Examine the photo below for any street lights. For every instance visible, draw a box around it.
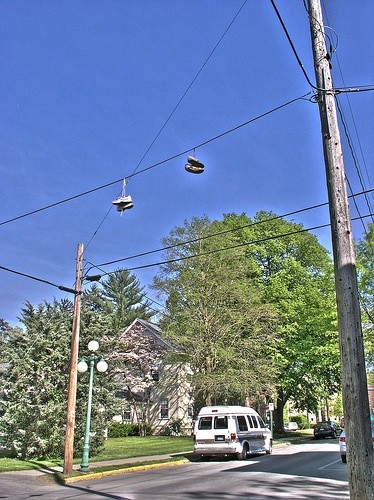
[77,339,108,472]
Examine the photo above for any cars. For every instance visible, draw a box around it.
[313,415,345,439]
[265,420,275,429]
[283,421,299,431]
[339,416,374,463]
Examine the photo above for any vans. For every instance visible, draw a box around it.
[193,405,274,462]
